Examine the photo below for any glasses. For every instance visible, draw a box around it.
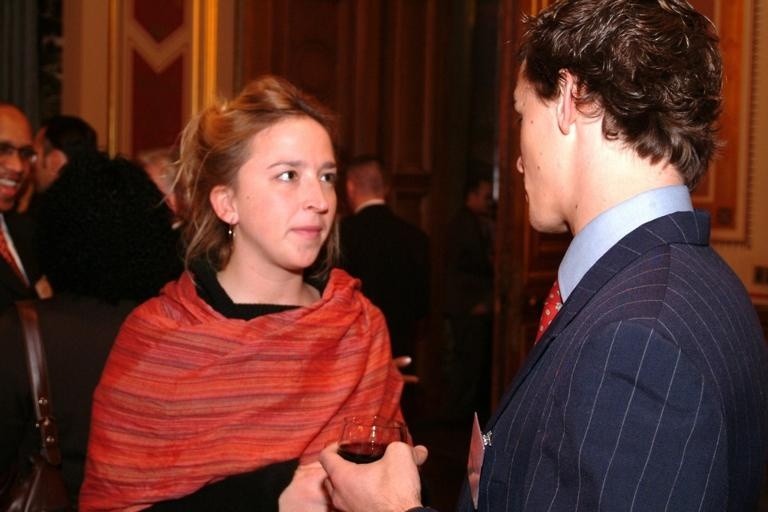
[0,140,41,166]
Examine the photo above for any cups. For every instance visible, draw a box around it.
[337,414,408,466]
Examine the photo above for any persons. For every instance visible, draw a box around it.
[307,154,434,403]
[0,100,205,512]
[316,0,765,512]
[77,76,415,512]
[441,182,495,431]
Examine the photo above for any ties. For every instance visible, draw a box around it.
[0,220,26,288]
[533,268,564,350]
